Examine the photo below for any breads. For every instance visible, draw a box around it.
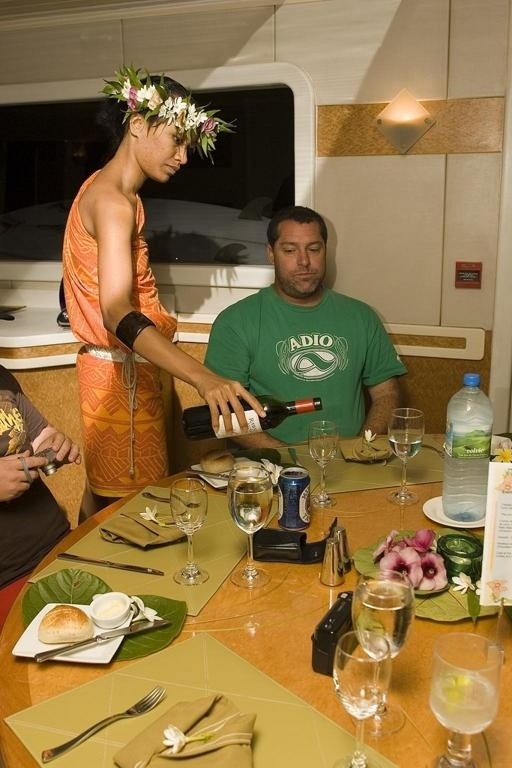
[38,604,93,643]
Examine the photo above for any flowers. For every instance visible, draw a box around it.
[99,62,240,166]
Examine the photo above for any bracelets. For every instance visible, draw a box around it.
[116,310,156,350]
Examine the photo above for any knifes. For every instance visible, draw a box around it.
[183,467,268,483]
[57,549,164,580]
[32,615,172,663]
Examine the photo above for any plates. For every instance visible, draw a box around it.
[422,493,484,530]
[10,601,135,665]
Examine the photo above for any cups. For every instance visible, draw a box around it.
[388,409,425,507]
[168,477,210,588]
[350,567,416,738]
[307,419,339,510]
[425,631,504,768]
[226,460,276,592]
[330,629,394,768]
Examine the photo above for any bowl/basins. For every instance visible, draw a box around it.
[86,591,133,629]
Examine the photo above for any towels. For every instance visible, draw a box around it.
[338,438,392,464]
[100,512,191,552]
[115,697,256,768]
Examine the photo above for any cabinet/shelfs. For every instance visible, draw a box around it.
[0,304,179,530]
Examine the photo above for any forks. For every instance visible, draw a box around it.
[40,684,170,764]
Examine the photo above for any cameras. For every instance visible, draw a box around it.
[312,590,354,676]
[34,448,71,476]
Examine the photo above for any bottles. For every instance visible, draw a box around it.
[182,395,322,440]
[320,538,344,587]
[442,374,493,521]
[334,527,351,573]
[278,467,312,531]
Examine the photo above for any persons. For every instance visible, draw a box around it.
[63,75,266,527]
[204,207,409,451]
[0,363,82,632]
